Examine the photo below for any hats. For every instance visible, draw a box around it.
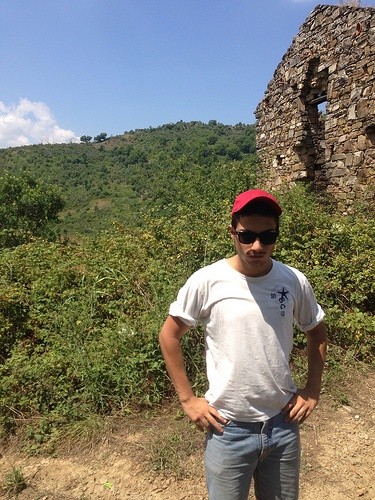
[230,190,282,216]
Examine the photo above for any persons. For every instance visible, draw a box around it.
[159,190,328,500]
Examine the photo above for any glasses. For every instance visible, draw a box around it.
[235,230,278,245]
